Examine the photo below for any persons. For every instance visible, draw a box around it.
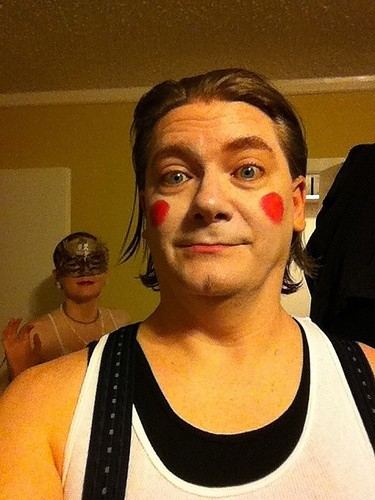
[0,232,132,385]
[0,69,375,500]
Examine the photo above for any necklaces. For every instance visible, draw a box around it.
[60,298,105,345]
